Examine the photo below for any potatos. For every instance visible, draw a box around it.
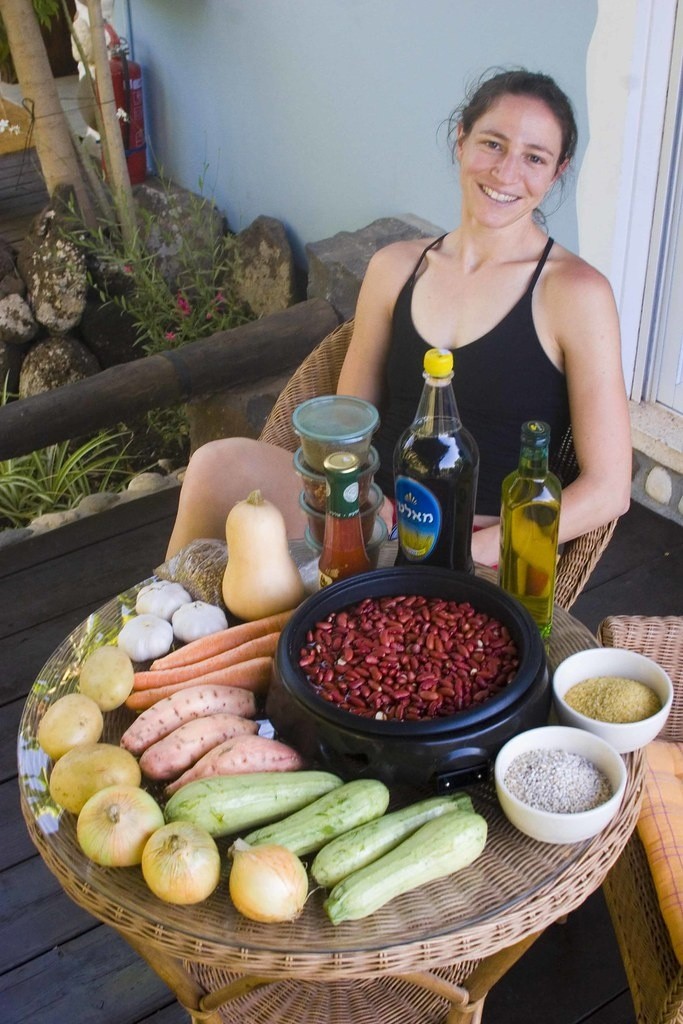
[122,684,300,794]
[39,647,141,813]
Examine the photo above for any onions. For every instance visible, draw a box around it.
[77,785,309,923]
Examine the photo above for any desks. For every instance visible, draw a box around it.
[12,530,644,1024]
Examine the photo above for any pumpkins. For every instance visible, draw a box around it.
[222,489,303,622]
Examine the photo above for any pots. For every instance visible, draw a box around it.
[264,566,551,812]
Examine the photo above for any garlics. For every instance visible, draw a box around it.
[117,580,227,661]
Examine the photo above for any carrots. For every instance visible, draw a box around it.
[126,609,295,710]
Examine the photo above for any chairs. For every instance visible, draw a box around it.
[593,614,683,1024]
[249,311,618,613]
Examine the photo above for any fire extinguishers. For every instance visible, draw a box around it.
[100,17,147,188]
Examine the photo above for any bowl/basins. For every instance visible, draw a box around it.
[298,483,383,546]
[291,395,379,472]
[552,647,674,753]
[293,445,378,512]
[494,726,628,844]
[304,514,388,571]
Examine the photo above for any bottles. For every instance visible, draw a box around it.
[394,349,479,571]
[314,452,372,591]
[498,421,563,636]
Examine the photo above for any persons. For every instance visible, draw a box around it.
[163,65,635,576]
